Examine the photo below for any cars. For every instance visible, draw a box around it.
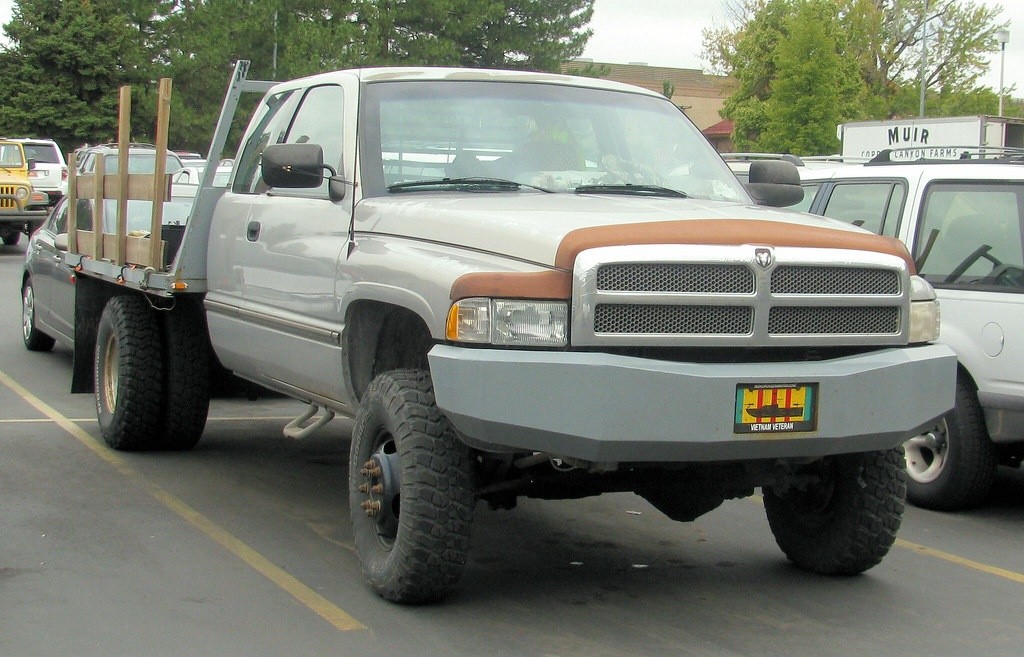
[0,133,236,247]
[18,183,202,354]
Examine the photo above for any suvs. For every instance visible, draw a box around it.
[712,149,1023,514]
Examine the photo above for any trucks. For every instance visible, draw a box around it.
[835,113,1024,162]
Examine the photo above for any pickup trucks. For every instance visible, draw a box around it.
[59,57,961,608]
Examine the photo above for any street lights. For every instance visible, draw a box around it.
[996,28,1010,117]
